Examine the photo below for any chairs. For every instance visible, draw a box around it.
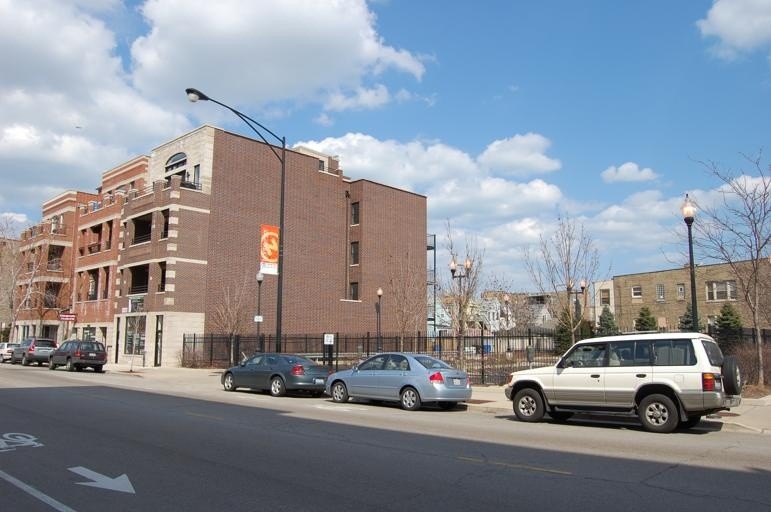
[373,356,410,370]
[588,345,650,366]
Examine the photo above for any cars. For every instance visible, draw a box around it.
[0,337,107,373]
[221,352,472,411]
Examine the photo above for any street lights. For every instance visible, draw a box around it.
[377,286,383,352]
[504,293,512,352]
[256,272,264,354]
[185,88,286,352]
[451,258,471,334]
[567,280,585,341]
[681,194,699,332]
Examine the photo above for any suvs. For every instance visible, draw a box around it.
[505,332,742,433]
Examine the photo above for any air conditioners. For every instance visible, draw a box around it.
[676,295,684,301]
